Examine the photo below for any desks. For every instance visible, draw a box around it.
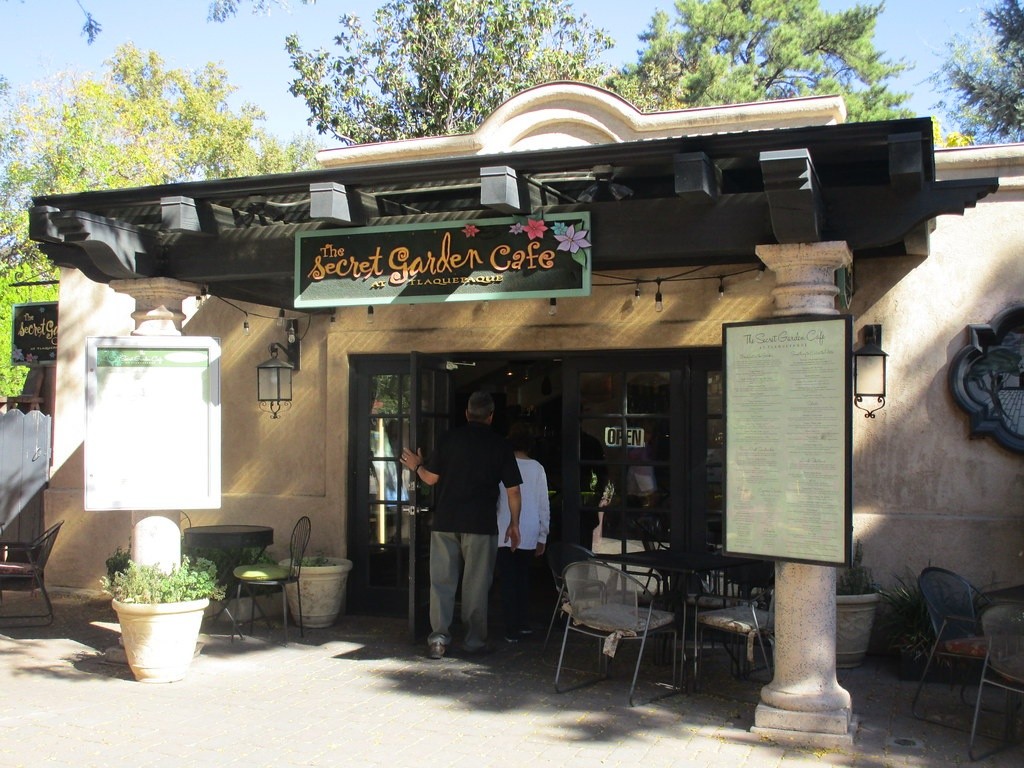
[587,548,762,697]
[185,523,274,639]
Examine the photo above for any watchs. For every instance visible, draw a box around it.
[415,464,422,471]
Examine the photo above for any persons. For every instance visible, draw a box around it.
[546,396,607,553]
[497,423,550,640]
[630,401,670,506]
[400,392,523,659]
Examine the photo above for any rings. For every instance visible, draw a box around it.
[405,458,407,460]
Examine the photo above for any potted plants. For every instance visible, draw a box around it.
[100,548,228,682]
[881,558,943,682]
[279,556,352,628]
[835,542,880,670]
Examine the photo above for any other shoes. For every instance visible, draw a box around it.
[502,629,519,643]
[472,639,496,659]
[518,624,534,635]
[429,641,449,658]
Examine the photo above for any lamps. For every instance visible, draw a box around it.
[256,338,300,419]
[852,324,888,417]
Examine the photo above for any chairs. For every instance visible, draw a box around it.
[217,515,312,647]
[0,518,66,629]
[544,540,775,708]
[911,567,1024,768]
